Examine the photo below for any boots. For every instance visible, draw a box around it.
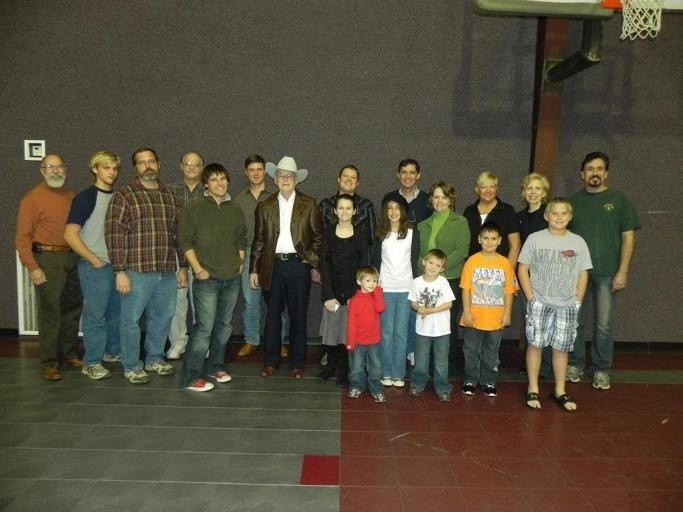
[318,343,348,388]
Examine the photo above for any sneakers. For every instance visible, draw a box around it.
[462,383,477,396]
[479,382,497,397]
[592,370,611,390]
[166,347,186,359]
[565,364,585,383]
[185,379,215,393]
[43,353,176,384]
[345,375,451,403]
[209,370,232,383]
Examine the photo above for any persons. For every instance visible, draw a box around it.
[105,148,175,384]
[464,171,523,369]
[517,196,593,411]
[567,152,642,389]
[65,151,124,380]
[407,249,456,403]
[415,181,471,381]
[236,154,435,404]
[166,151,211,360]
[176,164,247,391]
[13,153,83,381]
[458,222,520,397]
[515,172,552,379]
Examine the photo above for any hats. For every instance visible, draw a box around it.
[265,155,309,184]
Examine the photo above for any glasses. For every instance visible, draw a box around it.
[43,164,67,171]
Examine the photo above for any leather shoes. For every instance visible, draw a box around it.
[291,367,304,378]
[262,366,275,377]
[280,345,288,358]
[237,343,257,357]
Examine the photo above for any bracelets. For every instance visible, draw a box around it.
[195,270,205,275]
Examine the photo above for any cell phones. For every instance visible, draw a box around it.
[324,301,340,313]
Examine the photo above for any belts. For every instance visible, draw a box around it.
[31,242,70,253]
[273,252,301,261]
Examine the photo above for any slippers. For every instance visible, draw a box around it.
[525,390,543,410]
[553,391,576,413]
